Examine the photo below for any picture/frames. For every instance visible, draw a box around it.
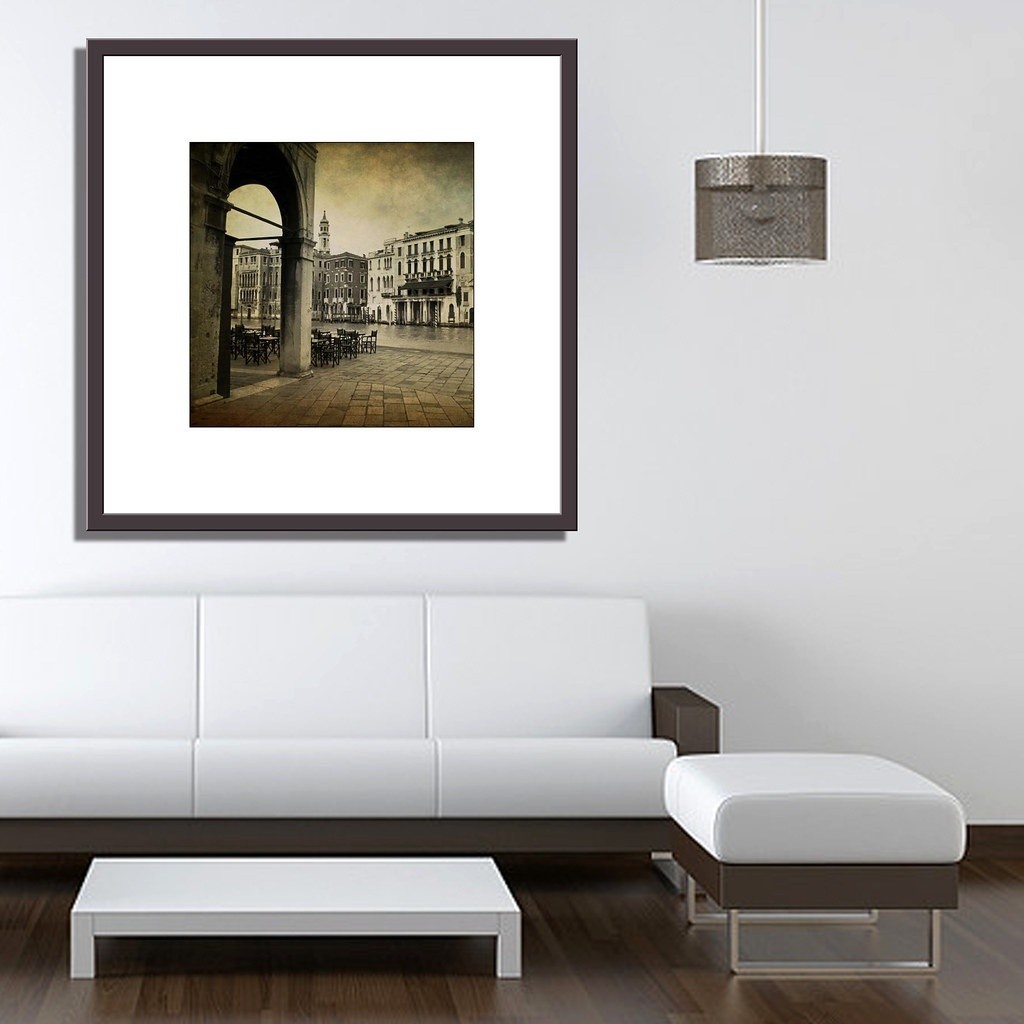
[85,38,583,533]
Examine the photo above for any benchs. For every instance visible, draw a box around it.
[0,592,722,898]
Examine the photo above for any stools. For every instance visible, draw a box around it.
[664,751,968,976]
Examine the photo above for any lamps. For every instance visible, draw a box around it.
[696,0,830,265]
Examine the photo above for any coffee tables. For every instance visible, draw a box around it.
[67,856,523,980]
